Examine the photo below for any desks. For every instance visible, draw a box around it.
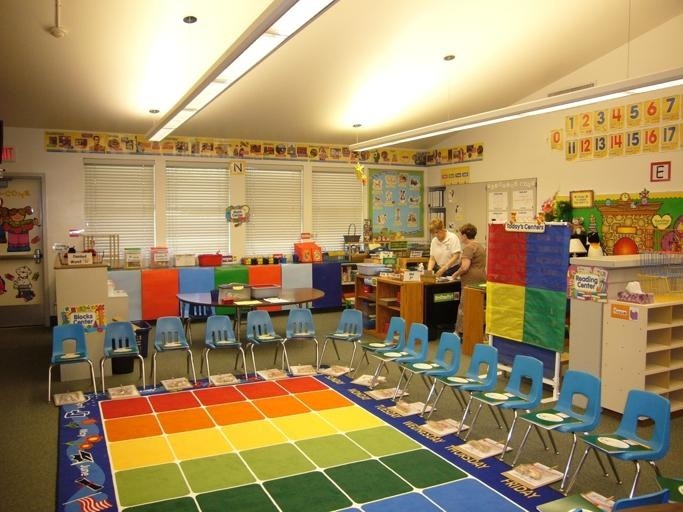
[176,287,325,346]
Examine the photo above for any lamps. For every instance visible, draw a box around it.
[349,0,683,152]
[146,0,338,141]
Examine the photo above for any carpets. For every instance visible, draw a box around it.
[55,366,611,512]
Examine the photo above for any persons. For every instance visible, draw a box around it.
[453,224,486,337]
[427,216,461,277]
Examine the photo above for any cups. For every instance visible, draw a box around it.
[210,290,219,302]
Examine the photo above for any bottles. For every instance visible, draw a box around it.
[418,262,424,273]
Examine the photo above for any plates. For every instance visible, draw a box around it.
[437,276,460,281]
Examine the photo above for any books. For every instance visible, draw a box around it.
[419,418,513,461]
[55,351,87,363]
[500,462,564,490]
[361,340,414,363]
[350,374,387,388]
[158,342,188,352]
[256,334,284,343]
[327,333,355,340]
[470,391,583,430]
[160,376,194,392]
[109,346,140,356]
[320,364,355,377]
[108,384,141,399]
[363,387,409,401]
[256,368,289,382]
[289,364,317,376]
[377,401,438,418]
[53,391,86,408]
[215,340,242,347]
[291,332,313,337]
[208,373,241,386]
[579,433,655,454]
[536,492,614,512]
[398,359,484,387]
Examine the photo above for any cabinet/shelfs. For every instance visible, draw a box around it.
[604,301,683,421]
[0,171,51,328]
[354,276,424,341]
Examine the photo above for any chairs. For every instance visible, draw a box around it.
[320,309,369,372]
[244,309,292,379]
[564,389,673,499]
[48,316,196,405]
[283,307,320,370]
[399,324,605,512]
[612,488,669,512]
[199,315,248,384]
[353,315,409,391]
